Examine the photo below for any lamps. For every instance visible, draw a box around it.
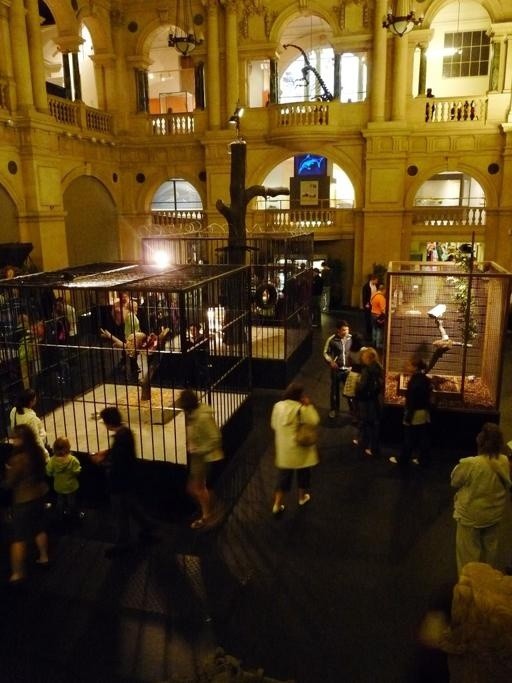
[382,0,424,37]
[168,0,205,56]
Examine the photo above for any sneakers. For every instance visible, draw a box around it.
[2,509,162,588]
[328,407,426,468]
[187,491,310,536]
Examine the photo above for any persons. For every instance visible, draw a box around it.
[323,322,450,467]
[271,385,320,513]
[13,298,247,405]
[93,408,156,559]
[425,87,436,122]
[249,260,330,328]
[362,275,387,349]
[2,388,82,585]
[419,422,511,681]
[176,390,223,532]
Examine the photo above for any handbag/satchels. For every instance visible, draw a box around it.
[292,405,318,447]
